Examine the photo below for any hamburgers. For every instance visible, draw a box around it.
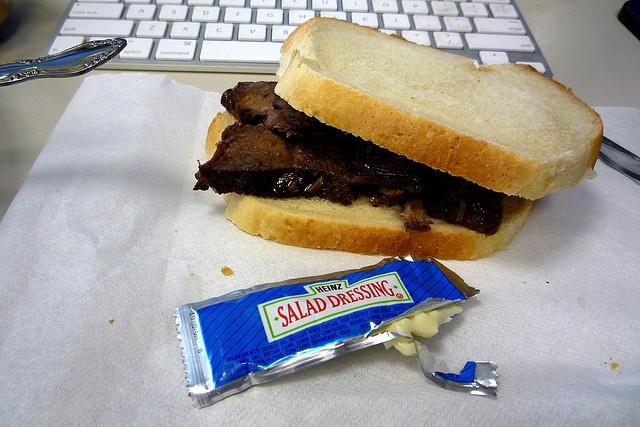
[195,16,602,258]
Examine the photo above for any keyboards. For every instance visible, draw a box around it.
[42,0,553,80]
